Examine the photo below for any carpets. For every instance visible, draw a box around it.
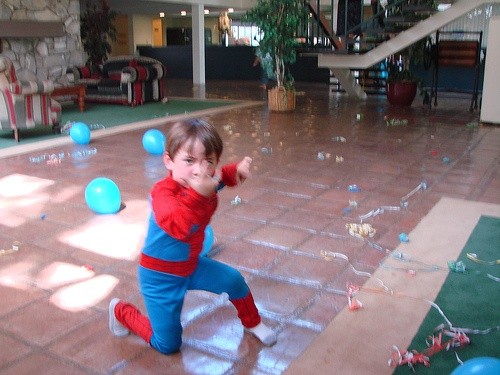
[0,96,267,158]
[281,195,500,375]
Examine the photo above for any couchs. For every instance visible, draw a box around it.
[72,56,167,107]
[0,54,62,143]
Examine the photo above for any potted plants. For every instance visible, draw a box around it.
[372,0,437,108]
[241,0,311,112]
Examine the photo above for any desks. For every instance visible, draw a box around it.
[50,85,87,112]
